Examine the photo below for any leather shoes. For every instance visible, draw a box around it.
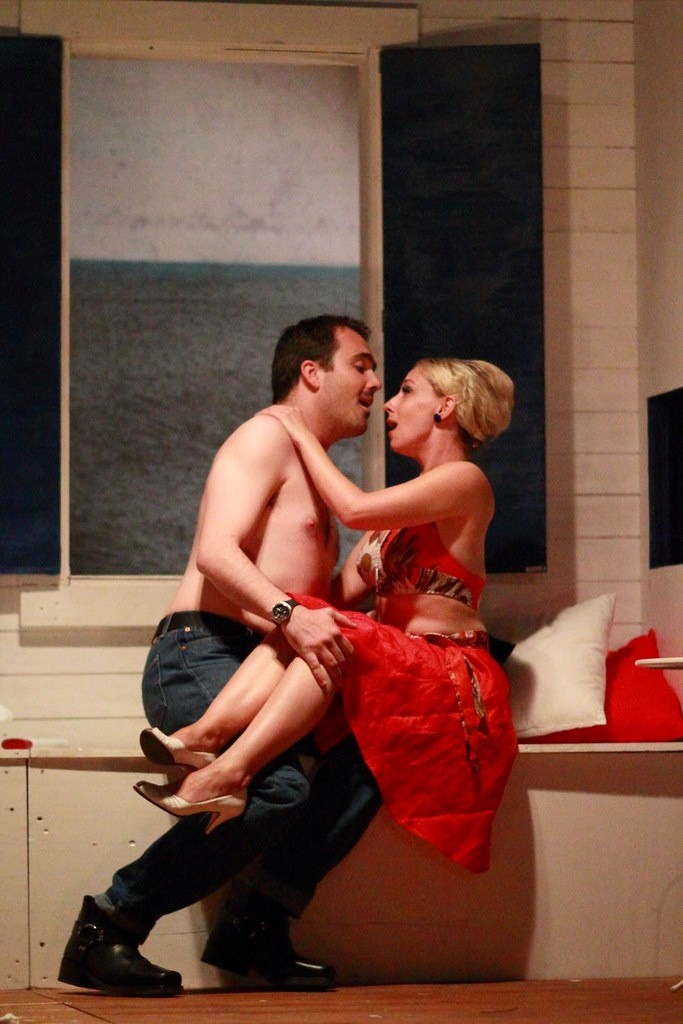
[200,880,337,988]
[57,895,185,995]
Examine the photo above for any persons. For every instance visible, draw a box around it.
[132,353,514,869]
[57,315,383,997]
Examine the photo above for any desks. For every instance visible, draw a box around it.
[0,744,683,991]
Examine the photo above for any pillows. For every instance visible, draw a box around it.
[521,628,683,744]
[503,592,615,739]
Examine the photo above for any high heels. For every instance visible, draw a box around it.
[133,780,247,834]
[140,726,219,771]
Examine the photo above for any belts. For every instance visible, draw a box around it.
[155,611,252,647]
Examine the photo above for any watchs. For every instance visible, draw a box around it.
[272,598,298,627]
